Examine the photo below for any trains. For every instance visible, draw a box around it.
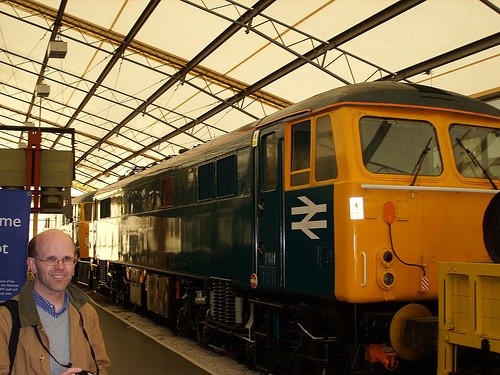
[41,81,499,375]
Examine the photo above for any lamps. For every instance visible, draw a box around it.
[18,135,27,149]
[46,23,67,59]
[35,70,50,97]
[25,112,35,133]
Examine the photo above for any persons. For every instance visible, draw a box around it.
[0,228,111,375]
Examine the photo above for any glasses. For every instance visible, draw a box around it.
[34,257,78,266]
